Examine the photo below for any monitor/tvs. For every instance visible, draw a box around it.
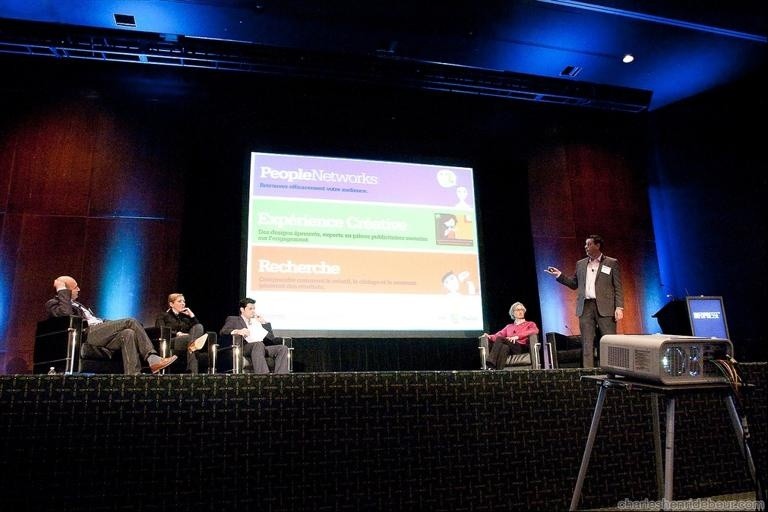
[653,295,732,342]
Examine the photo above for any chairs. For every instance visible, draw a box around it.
[34,319,293,374]
[477,333,556,370]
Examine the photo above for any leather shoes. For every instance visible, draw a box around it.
[150,355,177,374]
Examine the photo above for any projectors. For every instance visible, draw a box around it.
[599,331,737,386]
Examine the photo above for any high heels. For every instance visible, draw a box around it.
[188,333,208,355]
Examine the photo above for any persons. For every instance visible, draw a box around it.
[477,301,539,370]
[542,234,625,368]
[219,297,289,375]
[46,275,178,375]
[156,292,210,375]
